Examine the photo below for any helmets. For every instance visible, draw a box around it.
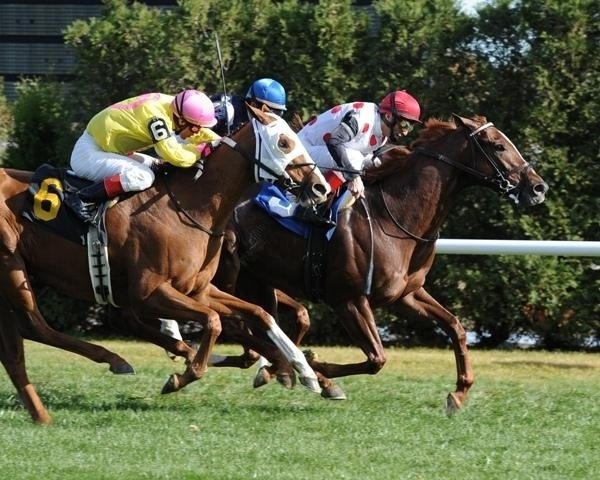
[379,90,422,123]
[246,78,287,111]
[172,90,218,128]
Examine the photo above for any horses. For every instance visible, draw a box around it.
[0,105,553,423]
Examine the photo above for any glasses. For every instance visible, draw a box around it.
[395,117,414,133]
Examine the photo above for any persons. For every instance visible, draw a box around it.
[62,89,219,220]
[296,91,422,218]
[163,76,287,181]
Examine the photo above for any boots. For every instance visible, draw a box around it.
[64,178,109,228]
[292,188,335,225]
